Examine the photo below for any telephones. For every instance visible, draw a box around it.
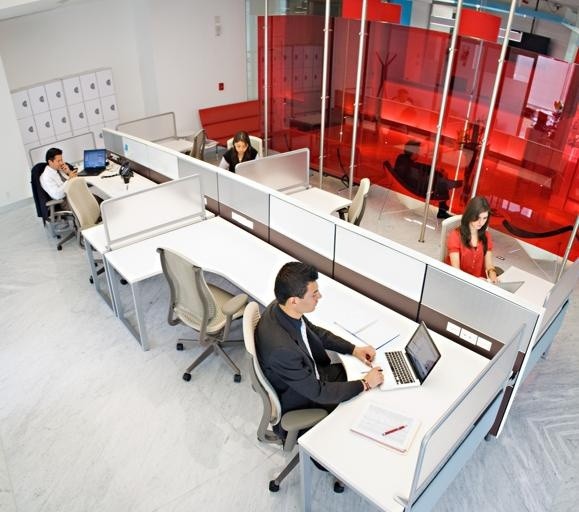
[119,160,134,177]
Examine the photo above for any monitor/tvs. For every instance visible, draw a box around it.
[505,29,550,69]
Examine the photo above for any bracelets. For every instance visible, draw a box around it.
[362,378,371,391]
[487,268,497,276]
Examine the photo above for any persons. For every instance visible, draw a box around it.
[446,197,501,284]
[254,262,384,472]
[393,141,463,218]
[391,89,414,105]
[218,131,261,173]
[39,148,79,221]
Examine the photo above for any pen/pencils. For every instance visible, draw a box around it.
[362,369,383,374]
[382,425,408,436]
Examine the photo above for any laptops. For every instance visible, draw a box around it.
[371,321,441,391]
[77,149,106,176]
[490,280,524,293]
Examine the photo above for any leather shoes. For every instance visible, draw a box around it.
[449,180,462,188]
[437,210,452,219]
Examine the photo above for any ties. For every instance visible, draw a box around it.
[301,320,320,380]
[57,170,67,182]
